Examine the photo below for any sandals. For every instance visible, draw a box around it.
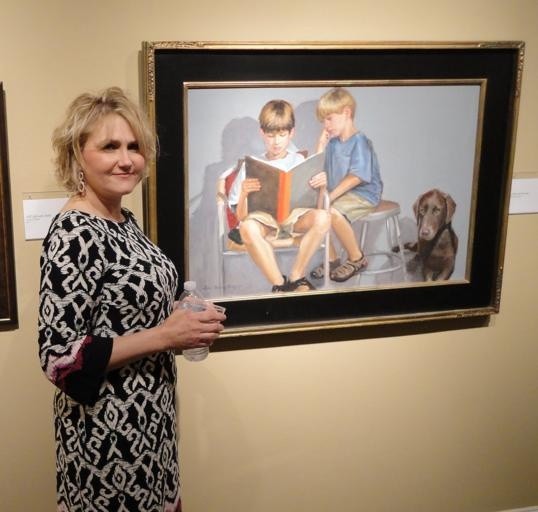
[330,253,368,282]
[272,273,315,293]
[311,259,340,279]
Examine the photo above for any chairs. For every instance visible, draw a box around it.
[214,160,331,297]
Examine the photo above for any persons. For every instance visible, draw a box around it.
[36,84,226,511]
[309,87,384,283]
[227,98,332,294]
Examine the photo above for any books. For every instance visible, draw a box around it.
[242,150,327,226]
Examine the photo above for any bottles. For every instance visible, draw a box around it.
[179,282,212,360]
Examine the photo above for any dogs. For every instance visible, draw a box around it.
[391,188,459,282]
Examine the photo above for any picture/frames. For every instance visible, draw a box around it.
[142,40,525,355]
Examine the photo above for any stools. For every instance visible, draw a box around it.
[339,201,408,286]
